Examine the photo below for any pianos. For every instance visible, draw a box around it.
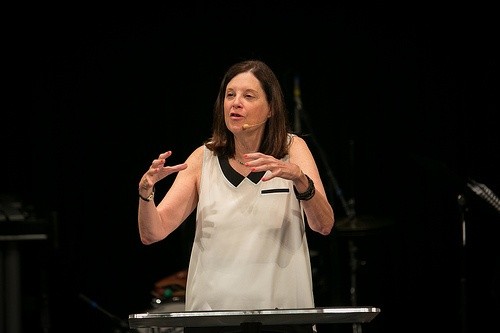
[128,306,381,328]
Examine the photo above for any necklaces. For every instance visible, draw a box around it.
[227,152,244,165]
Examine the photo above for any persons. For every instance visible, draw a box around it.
[137,58,335,333]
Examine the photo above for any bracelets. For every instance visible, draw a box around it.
[293,174,316,201]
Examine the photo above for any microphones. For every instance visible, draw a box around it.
[243,118,267,128]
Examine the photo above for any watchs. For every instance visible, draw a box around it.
[139,187,156,202]
